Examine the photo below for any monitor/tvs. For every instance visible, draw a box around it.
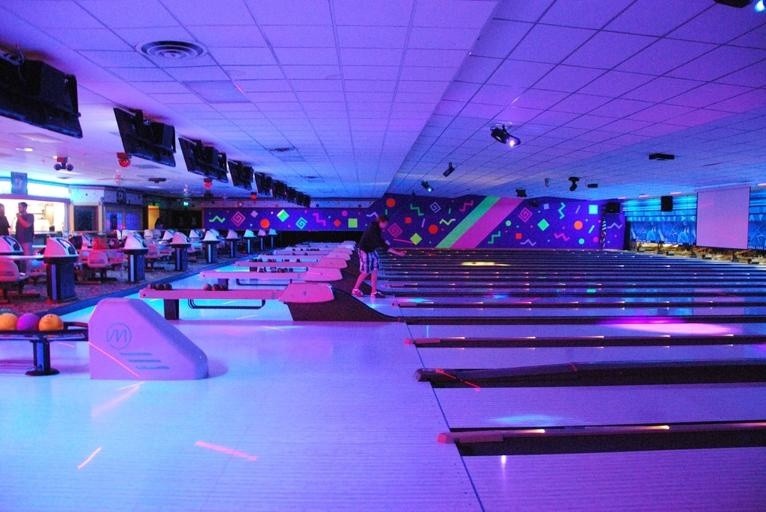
[177,135,228,184]
[112,105,177,170]
[227,157,312,210]
[0,51,84,140]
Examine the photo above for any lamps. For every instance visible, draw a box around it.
[568,176,579,191]
[420,180,434,193]
[491,124,521,147]
[443,162,455,177]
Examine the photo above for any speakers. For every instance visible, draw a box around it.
[661,196,673,212]
[606,202,621,214]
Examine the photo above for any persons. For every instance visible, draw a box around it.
[0,203,11,235]
[351,214,407,299]
[16,201,35,273]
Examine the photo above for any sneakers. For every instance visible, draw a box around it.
[351,288,364,296]
[370,290,386,298]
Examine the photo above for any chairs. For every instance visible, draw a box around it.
[0,229,246,299]
